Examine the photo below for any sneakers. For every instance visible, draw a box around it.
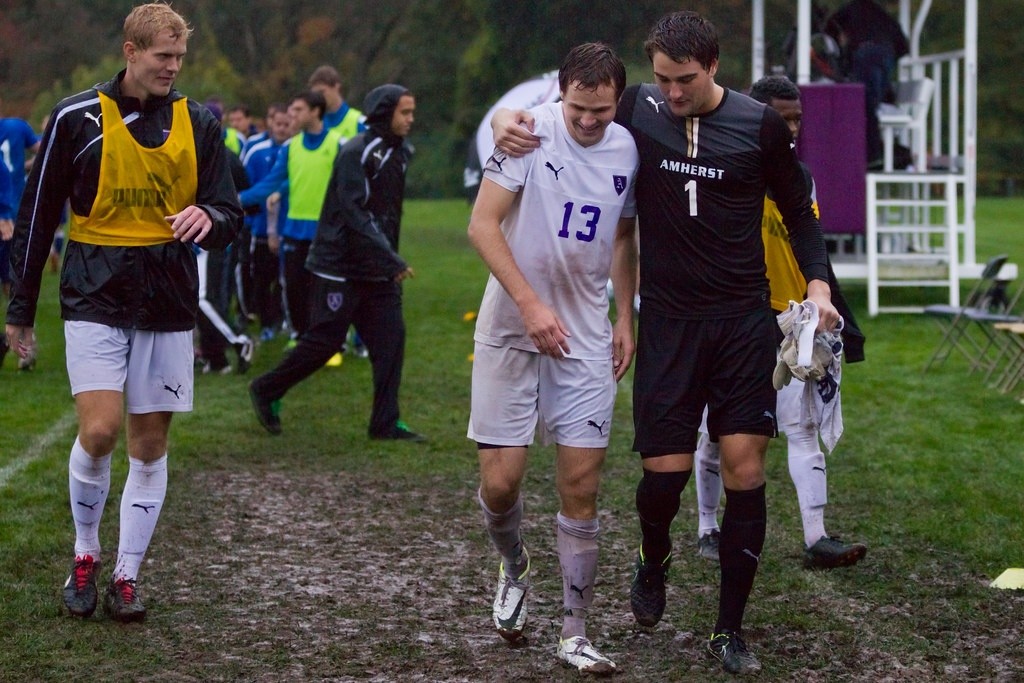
[493,548,531,640]
[803,535,865,570]
[697,528,722,563]
[249,377,283,434]
[103,575,146,621]
[556,635,618,676]
[631,545,672,628]
[63,559,98,616]
[706,629,763,677]
[371,420,429,443]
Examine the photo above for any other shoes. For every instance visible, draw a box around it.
[188,321,370,377]
[18,334,38,374]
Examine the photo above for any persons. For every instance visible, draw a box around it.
[0,115,67,373]
[249,85,422,438]
[489,12,842,679]
[467,43,642,675]
[691,73,870,569]
[194,67,372,379]
[5,0,246,619]
[824,0,908,169]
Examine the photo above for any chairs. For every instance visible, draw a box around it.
[873,78,934,170]
[916,252,1009,376]
[990,319,1024,396]
[964,274,1024,383]
